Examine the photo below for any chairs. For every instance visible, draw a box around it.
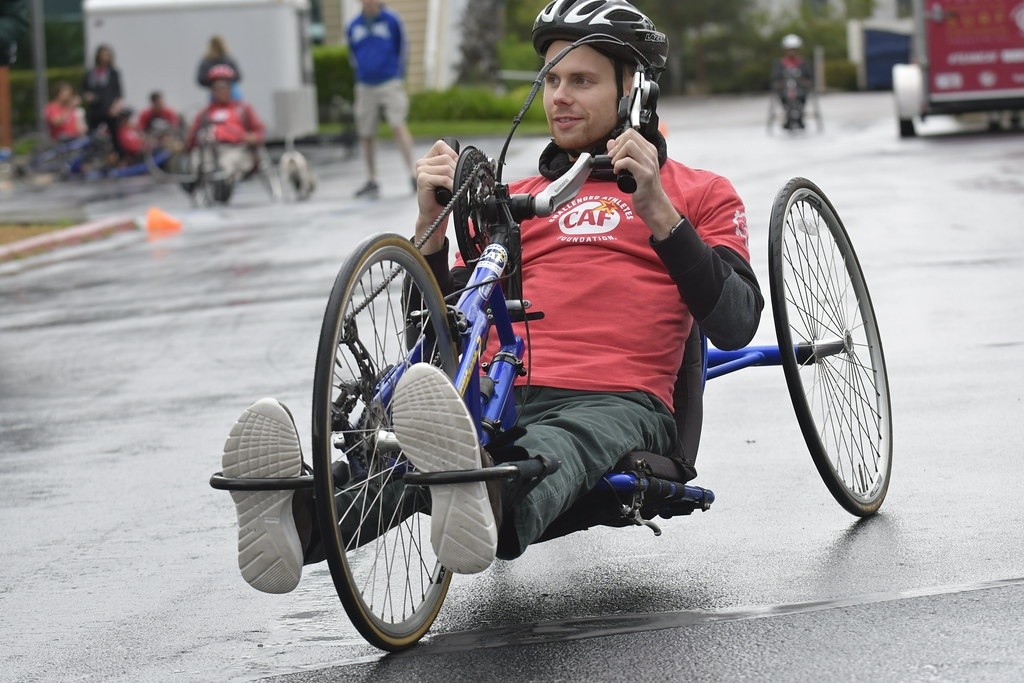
[539,315,712,543]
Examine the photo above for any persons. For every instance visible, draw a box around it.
[117,111,157,155]
[776,35,813,129]
[82,45,127,165]
[198,37,242,102]
[45,82,88,144]
[347,0,420,196]
[222,0,765,596]
[180,63,266,202]
[136,94,181,134]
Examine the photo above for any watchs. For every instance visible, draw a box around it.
[672,219,684,235]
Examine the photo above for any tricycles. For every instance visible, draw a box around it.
[761,68,826,135]
[147,111,316,208]
[204,33,890,655]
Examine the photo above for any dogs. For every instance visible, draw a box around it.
[276,132,316,204]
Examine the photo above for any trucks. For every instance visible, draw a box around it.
[893,0,1024,136]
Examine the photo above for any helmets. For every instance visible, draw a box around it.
[533,0,670,84]
[208,65,236,80]
[780,35,804,49]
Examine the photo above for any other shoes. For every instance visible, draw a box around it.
[392,363,507,575]
[222,399,317,594]
[354,181,380,196]
[411,177,417,192]
